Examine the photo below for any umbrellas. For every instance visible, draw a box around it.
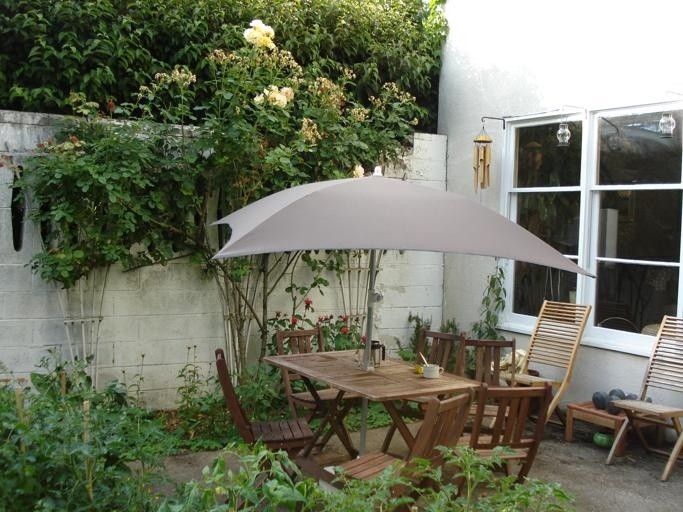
[208,166,599,367]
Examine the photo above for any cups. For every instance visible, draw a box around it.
[423,364,445,378]
[371,340,385,368]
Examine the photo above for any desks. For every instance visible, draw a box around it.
[565,403,666,456]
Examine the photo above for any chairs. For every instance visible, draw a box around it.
[215,348,314,512]
[275,323,359,458]
[336,386,473,497]
[455,380,551,498]
[464,337,516,418]
[380,330,466,454]
[607,315,683,481]
[492,299,592,424]
